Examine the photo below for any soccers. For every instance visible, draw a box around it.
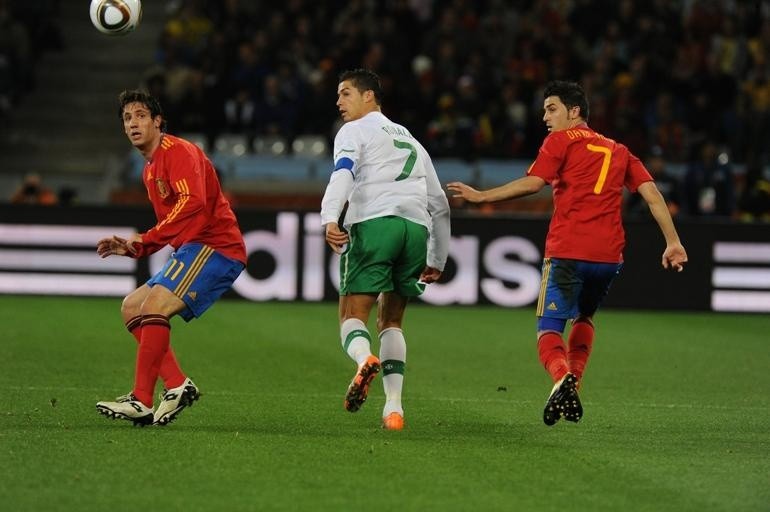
[90,0,142,36]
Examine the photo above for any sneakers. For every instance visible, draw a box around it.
[152,378,199,427]
[345,355,381,413]
[96,391,154,427]
[563,387,583,424]
[382,412,403,429]
[543,372,579,426]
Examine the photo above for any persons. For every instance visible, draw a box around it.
[318,69,450,429]
[95,91,249,426]
[1,0,770,226]
[445,80,688,426]
